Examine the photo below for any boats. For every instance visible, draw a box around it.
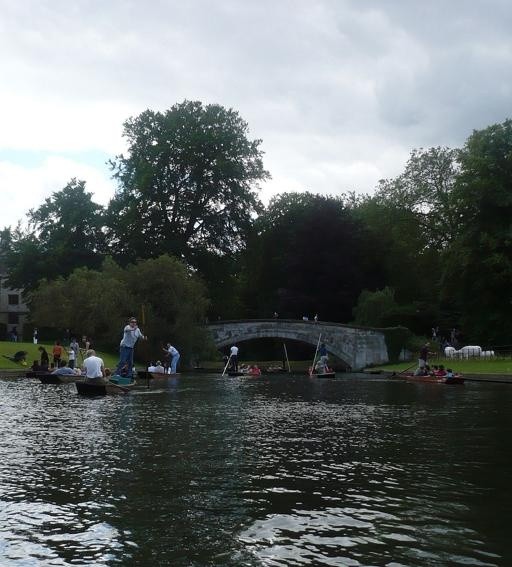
[405,374,464,384]
[26,370,182,396]
[226,365,335,378]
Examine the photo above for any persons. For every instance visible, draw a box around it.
[115,317,148,378]
[53,342,61,367]
[312,343,333,374]
[148,358,172,373]
[83,337,112,384]
[223,353,261,375]
[314,314,318,321]
[117,362,136,376]
[10,327,19,342]
[416,364,457,377]
[162,342,181,374]
[65,338,79,368]
[229,342,239,374]
[431,320,465,352]
[38,347,50,372]
[273,311,279,319]
[301,312,310,321]
[418,341,431,369]
[32,328,38,343]
[63,328,71,342]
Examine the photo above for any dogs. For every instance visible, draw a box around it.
[104,368,111,375]
[78,348,87,359]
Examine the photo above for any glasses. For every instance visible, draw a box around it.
[131,321,136,323]
